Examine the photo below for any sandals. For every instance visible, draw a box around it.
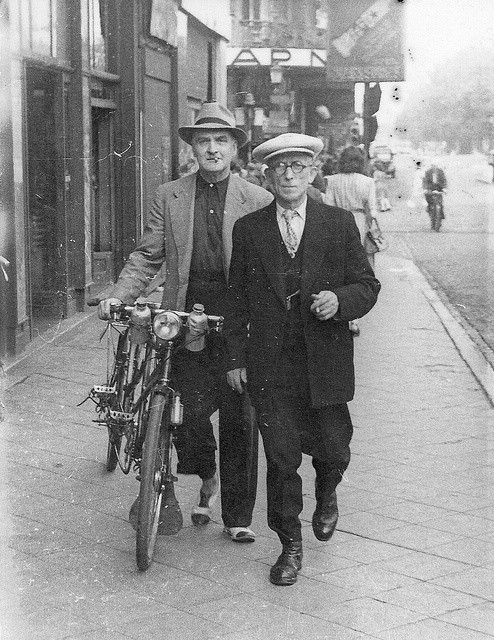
[351,323,360,337]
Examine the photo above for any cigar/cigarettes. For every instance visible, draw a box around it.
[215,157,218,162]
[314,299,320,313]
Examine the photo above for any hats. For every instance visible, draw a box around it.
[178,103,248,150]
[252,133,324,164]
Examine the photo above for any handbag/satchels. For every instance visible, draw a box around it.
[364,218,389,254]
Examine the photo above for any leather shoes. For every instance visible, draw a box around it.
[223,526,256,542]
[191,471,220,526]
[270,542,302,585]
[312,480,338,541]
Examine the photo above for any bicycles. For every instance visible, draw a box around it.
[78,297,224,574]
[424,185,445,232]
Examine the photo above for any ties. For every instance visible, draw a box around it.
[282,209,299,258]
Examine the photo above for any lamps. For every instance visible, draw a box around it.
[267,60,287,94]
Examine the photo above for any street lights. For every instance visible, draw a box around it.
[235,91,256,164]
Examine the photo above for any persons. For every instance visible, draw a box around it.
[373,164,391,212]
[96,103,275,543]
[423,162,447,219]
[222,132,380,585]
[324,146,378,337]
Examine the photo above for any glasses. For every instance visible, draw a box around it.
[268,161,312,175]
